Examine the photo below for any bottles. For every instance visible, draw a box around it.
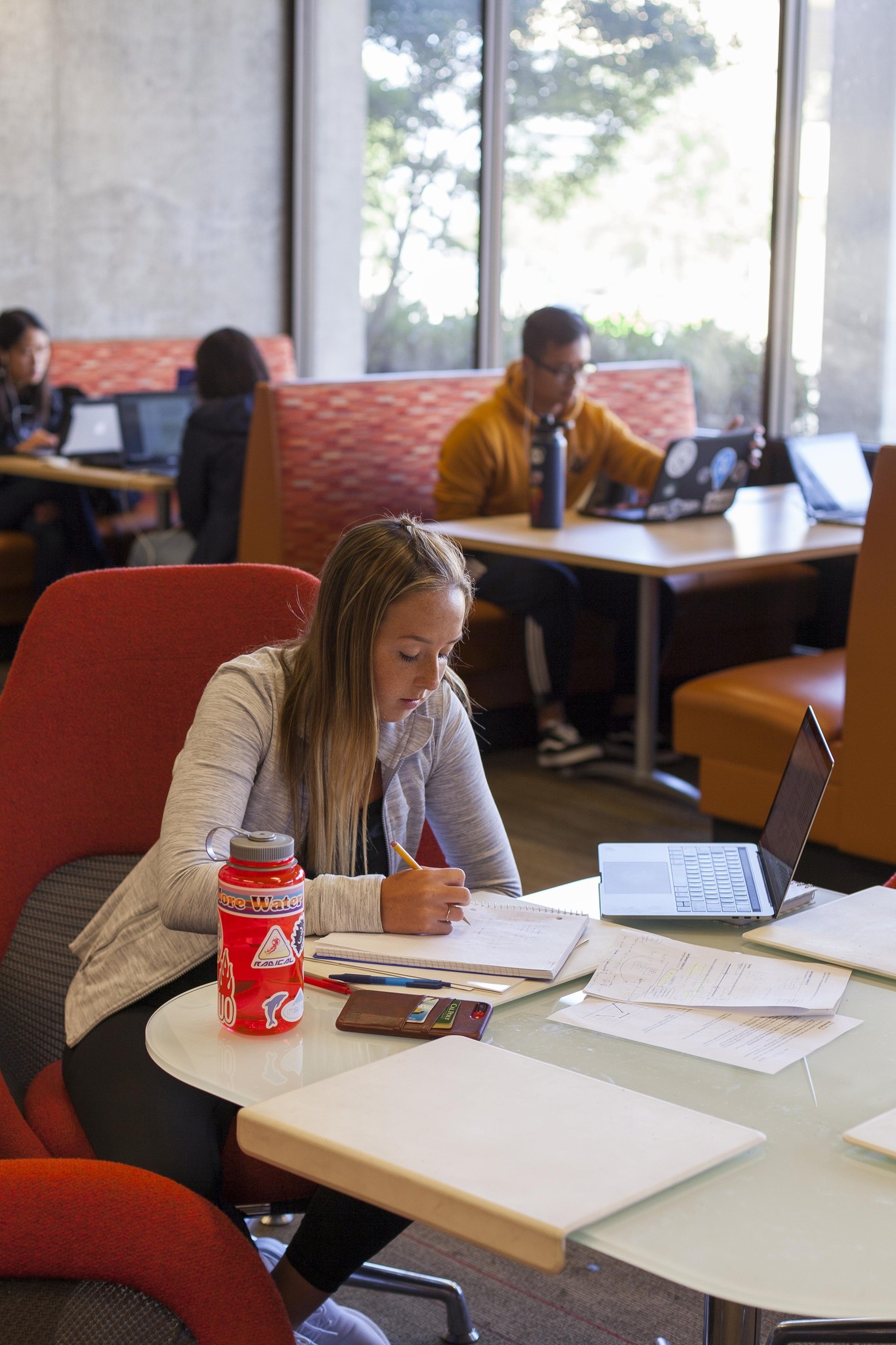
[528,413,576,530]
[203,824,307,1038]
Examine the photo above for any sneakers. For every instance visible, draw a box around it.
[539,723,605,770]
[255,1236,390,1345]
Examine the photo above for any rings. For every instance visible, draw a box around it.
[444,904,453,924]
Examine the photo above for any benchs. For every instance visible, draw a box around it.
[0,336,895,895]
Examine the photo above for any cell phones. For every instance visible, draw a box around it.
[335,990,493,1042]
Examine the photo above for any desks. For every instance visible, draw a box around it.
[144,873,896,1345]
[0,452,178,528]
[426,482,864,807]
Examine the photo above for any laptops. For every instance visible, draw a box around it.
[599,705,835,922]
[785,432,873,526]
[578,430,758,524]
[46,389,199,478]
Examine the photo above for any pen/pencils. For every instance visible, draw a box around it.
[388,839,473,927]
[302,971,452,995]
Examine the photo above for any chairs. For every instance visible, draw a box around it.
[0,568,485,1345]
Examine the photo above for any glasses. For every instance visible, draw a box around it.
[532,356,594,379]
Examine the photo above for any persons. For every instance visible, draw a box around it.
[128,328,271,565]
[432,303,766,771]
[61,512,525,1344]
[2,309,122,568]
